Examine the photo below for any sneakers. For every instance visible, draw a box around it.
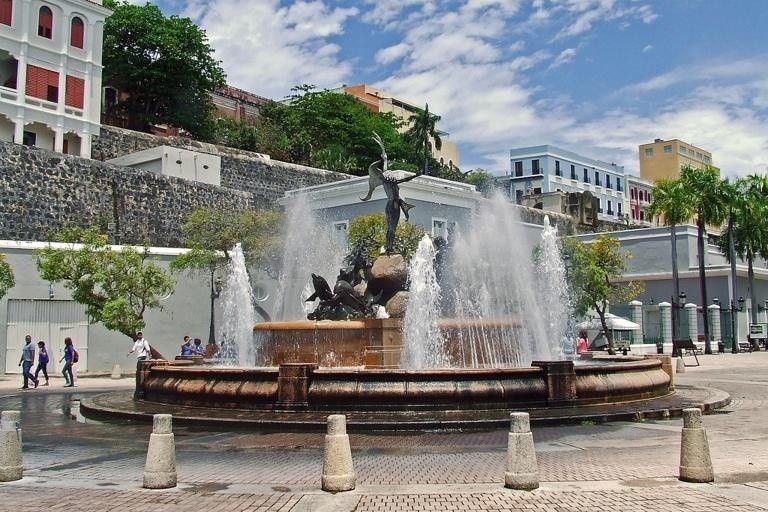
[64,382,74,387]
[23,385,28,388]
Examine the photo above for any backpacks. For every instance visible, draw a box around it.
[74,350,79,362]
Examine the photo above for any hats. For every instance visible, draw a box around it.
[37,341,45,345]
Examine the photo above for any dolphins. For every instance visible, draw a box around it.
[305,272,335,303]
[332,280,362,305]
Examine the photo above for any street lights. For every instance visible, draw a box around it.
[208,260,221,344]
[671,292,686,357]
[731,297,744,354]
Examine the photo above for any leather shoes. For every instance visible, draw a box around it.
[35,380,39,388]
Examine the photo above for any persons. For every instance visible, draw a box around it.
[127,331,152,363]
[181,336,195,355]
[560,329,575,354]
[59,337,74,387]
[576,330,588,354]
[19,335,39,389]
[31,341,49,385]
[191,339,208,355]
